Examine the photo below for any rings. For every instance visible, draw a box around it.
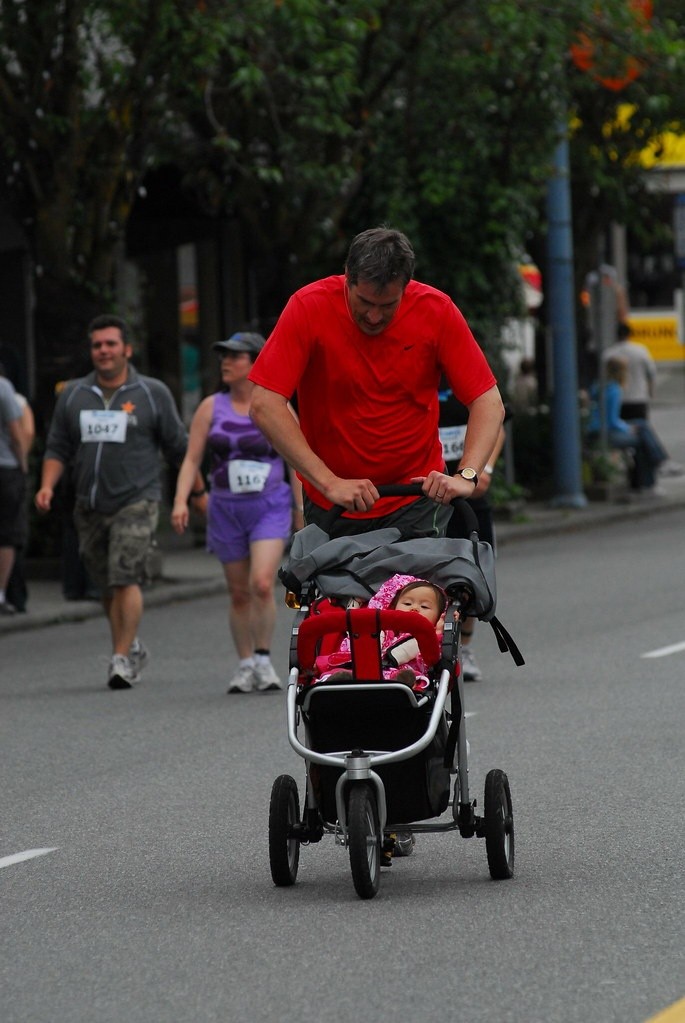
[436,493,443,498]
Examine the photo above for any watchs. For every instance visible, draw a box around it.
[454,468,478,488]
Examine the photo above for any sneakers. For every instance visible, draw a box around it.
[462,648,483,682]
[387,832,416,857]
[106,657,133,690]
[227,664,253,694]
[253,662,282,691]
[128,638,149,684]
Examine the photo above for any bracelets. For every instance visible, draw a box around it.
[192,487,208,496]
[295,505,303,510]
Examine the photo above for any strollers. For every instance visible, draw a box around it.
[267,482,516,900]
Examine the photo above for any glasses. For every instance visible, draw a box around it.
[216,353,250,362]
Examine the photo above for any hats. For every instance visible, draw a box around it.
[211,332,266,353]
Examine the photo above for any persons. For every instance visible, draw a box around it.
[0,378,34,617]
[436,371,507,682]
[36,317,211,689]
[172,333,306,692]
[513,322,662,496]
[316,581,460,686]
[246,229,506,856]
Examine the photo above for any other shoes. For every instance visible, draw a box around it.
[326,670,351,682]
[0,600,16,617]
[631,485,667,501]
[655,460,684,477]
[390,669,416,688]
